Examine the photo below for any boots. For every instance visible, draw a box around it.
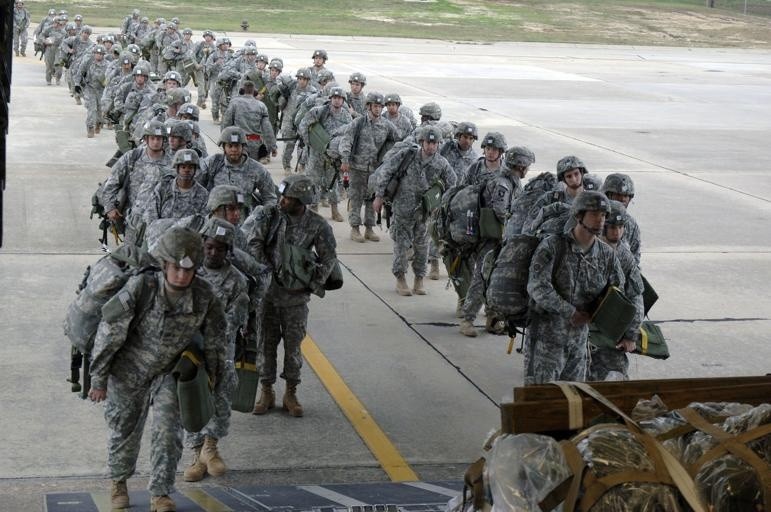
[20,50,26,57]
[364,226,380,241]
[456,296,468,318]
[198,435,226,478]
[394,272,413,296]
[414,275,427,295]
[110,479,130,510]
[87,124,95,138]
[282,382,304,417]
[212,110,220,125]
[460,320,478,336]
[150,495,179,512]
[184,447,207,482]
[95,120,102,134]
[197,99,201,108]
[351,226,365,243]
[15,49,19,56]
[201,99,206,109]
[331,203,343,222]
[430,259,440,281]
[46,76,52,85]
[254,381,276,415]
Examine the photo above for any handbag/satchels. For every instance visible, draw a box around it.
[476,206,514,240]
[630,321,670,360]
[278,240,344,291]
[422,178,446,216]
[230,340,259,414]
[176,340,216,434]
[307,119,330,155]
[592,284,638,346]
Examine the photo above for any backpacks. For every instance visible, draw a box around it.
[325,122,351,163]
[67,58,85,94]
[34,32,46,53]
[437,121,456,141]
[427,181,467,253]
[182,49,196,74]
[227,246,275,313]
[501,172,555,242]
[161,45,177,66]
[367,142,419,205]
[400,107,417,130]
[447,182,482,250]
[268,84,288,109]
[136,103,166,132]
[116,129,136,155]
[142,218,182,248]
[121,90,144,126]
[91,170,130,236]
[281,96,301,145]
[61,243,155,356]
[476,231,534,331]
[55,42,70,68]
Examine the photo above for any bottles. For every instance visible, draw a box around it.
[342,170,351,189]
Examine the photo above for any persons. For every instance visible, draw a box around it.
[32,3,647,509]
[13,0,30,57]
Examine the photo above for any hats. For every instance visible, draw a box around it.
[583,172,602,192]
[242,80,255,87]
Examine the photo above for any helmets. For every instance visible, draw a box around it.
[185,120,201,135]
[556,156,587,181]
[572,189,611,218]
[206,185,249,213]
[203,31,213,39]
[328,86,347,100]
[245,46,258,56]
[278,174,316,205]
[223,38,231,44]
[180,103,200,119]
[603,199,628,227]
[244,39,257,47]
[48,9,56,16]
[132,66,149,77]
[454,122,479,141]
[216,38,225,47]
[162,70,182,85]
[365,92,385,107]
[385,93,402,106]
[182,28,192,36]
[133,8,141,16]
[417,126,443,144]
[141,17,150,23]
[157,17,165,24]
[118,51,133,64]
[136,60,151,73]
[316,69,334,82]
[602,173,635,198]
[268,61,283,72]
[215,126,248,147]
[101,34,114,45]
[199,218,235,248]
[94,45,107,53]
[15,0,24,5]
[160,88,188,105]
[75,15,83,22]
[170,121,193,142]
[66,23,78,33]
[295,69,311,80]
[171,17,180,25]
[157,223,206,268]
[96,35,106,41]
[312,49,328,60]
[271,58,283,68]
[504,146,536,169]
[419,102,442,120]
[171,149,201,169]
[127,43,140,55]
[481,132,508,150]
[255,55,268,64]
[143,120,167,138]
[348,72,366,85]
[53,16,65,24]
[80,25,92,35]
[112,44,122,54]
[60,15,69,21]
[167,23,177,30]
